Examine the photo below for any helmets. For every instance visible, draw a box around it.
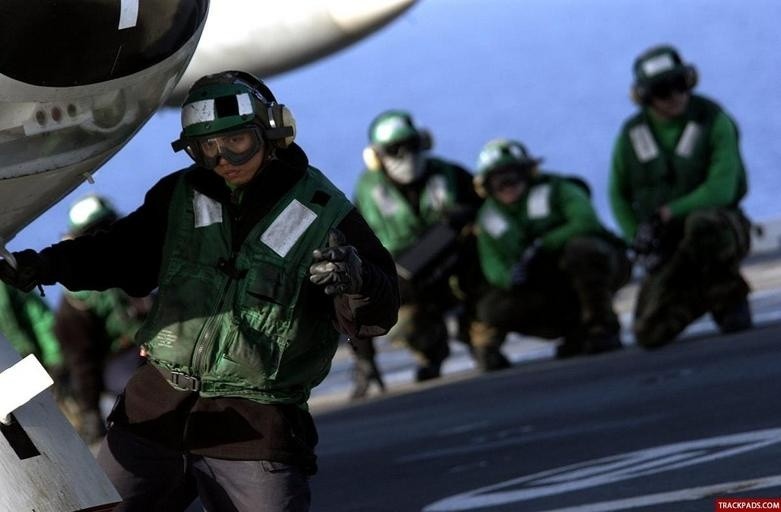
[473,139,544,200]
[171,69,297,150]
[362,109,431,173]
[629,45,698,107]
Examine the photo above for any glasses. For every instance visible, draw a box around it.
[649,74,691,100]
[385,137,433,157]
[489,165,533,186]
[177,123,266,170]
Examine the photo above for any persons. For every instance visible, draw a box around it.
[607,45,756,349]
[54,194,157,446]
[473,137,633,356]
[0,244,69,397]
[1,67,400,510]
[345,107,515,404]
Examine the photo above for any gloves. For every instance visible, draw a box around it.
[307,226,379,302]
[1,248,45,297]
